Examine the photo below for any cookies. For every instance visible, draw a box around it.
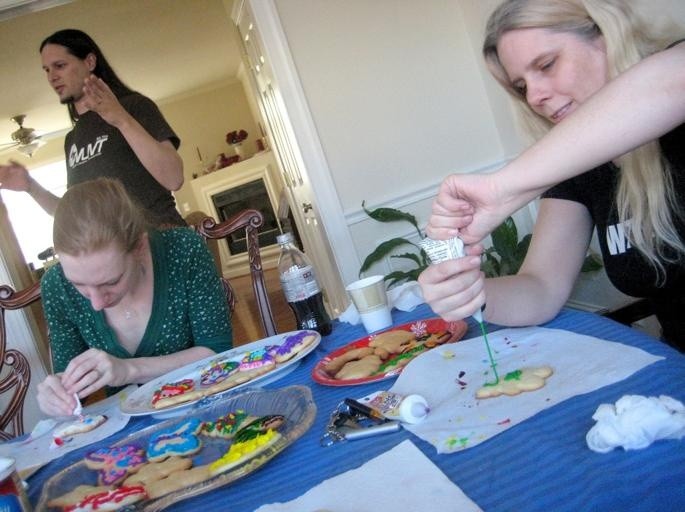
[48,329,552,512]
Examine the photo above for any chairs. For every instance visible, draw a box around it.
[605,296,654,327]
[0,209,279,444]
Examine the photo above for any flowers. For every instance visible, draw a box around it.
[226,129,248,146]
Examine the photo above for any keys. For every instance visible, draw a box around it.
[334,412,363,432]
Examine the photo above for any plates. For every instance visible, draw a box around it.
[313,315,468,386]
[36,384,317,512]
[117,329,320,421]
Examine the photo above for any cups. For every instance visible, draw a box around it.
[0,457,34,512]
[345,274,394,333]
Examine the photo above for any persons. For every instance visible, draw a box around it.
[30,177,237,422]
[415,0,685,362]
[1,27,189,225]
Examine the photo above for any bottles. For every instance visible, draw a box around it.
[276,232,333,335]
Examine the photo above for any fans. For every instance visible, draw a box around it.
[0,115,74,153]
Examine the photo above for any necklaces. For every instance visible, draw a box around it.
[118,294,133,321]
